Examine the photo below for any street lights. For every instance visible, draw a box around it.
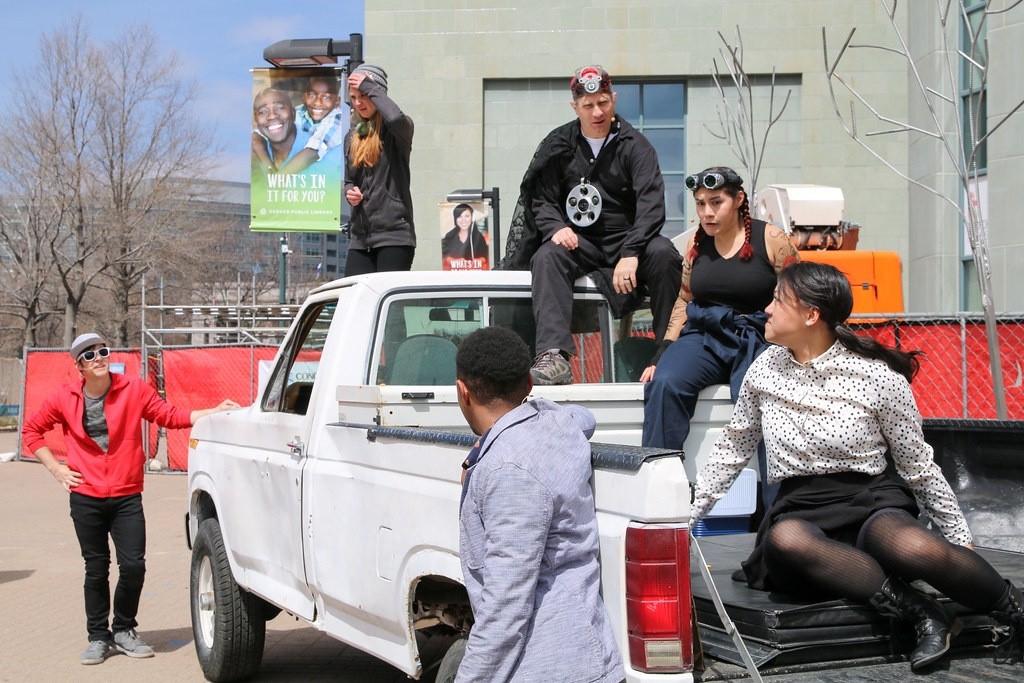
[447,188,500,267]
[263,33,364,236]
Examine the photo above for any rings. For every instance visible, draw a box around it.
[623,275,629,280]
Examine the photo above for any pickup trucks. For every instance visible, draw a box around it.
[185,271,1024,683]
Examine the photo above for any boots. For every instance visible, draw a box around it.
[974,579,1024,664]
[870,576,965,668]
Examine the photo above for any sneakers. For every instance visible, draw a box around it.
[110,628,154,658]
[530,350,573,385]
[81,640,110,664]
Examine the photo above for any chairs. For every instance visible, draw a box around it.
[612,336,658,383]
[386,333,458,386]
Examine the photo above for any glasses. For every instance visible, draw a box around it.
[76,347,111,363]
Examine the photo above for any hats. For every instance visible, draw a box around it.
[347,63,388,95]
[70,333,107,359]
[570,68,612,98]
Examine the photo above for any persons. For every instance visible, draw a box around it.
[686,260,1024,668]
[520,64,686,386]
[342,61,417,279]
[252,78,343,174]
[22,333,241,666]
[442,202,488,261]
[253,88,313,171]
[637,166,802,517]
[451,326,627,683]
